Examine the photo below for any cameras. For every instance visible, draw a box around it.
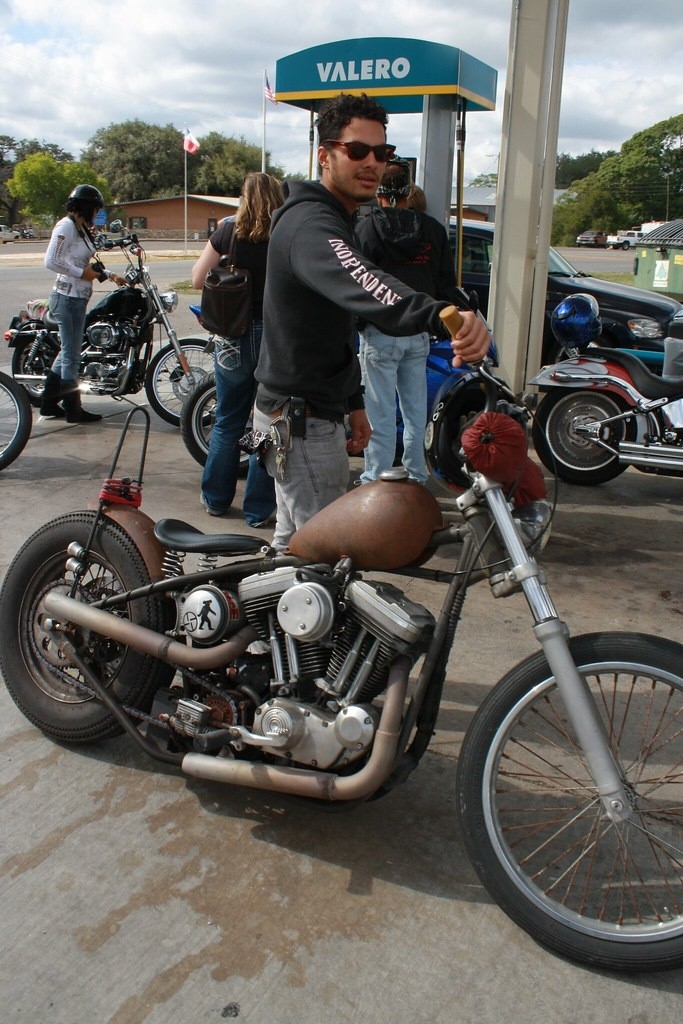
[92,262,107,283]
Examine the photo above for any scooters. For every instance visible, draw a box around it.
[1,371,34,472]
[527,292,683,487]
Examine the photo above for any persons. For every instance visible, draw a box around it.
[354,166,458,482]
[253,94,491,551]
[193,172,285,526]
[39,185,127,423]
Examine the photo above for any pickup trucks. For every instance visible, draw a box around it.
[606,231,648,250]
[576,230,607,249]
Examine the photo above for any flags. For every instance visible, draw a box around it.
[265,78,278,105]
[184,129,200,154]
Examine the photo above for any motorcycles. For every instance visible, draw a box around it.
[178,274,504,481]
[4,219,233,429]
[0,304,683,976]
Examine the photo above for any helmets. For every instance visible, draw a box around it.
[424,373,529,495]
[551,292,602,346]
[69,184,104,208]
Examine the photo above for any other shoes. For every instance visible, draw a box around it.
[200,492,221,516]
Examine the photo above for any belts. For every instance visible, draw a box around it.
[271,403,314,416]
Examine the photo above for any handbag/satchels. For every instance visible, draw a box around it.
[201,224,252,336]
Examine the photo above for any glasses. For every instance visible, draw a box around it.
[321,139,396,162]
[94,207,100,212]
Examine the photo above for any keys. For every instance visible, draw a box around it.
[276,454,286,480]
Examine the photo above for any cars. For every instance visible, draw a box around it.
[444,214,683,359]
[0,223,34,244]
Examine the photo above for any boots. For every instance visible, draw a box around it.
[40,371,65,416]
[61,379,102,422]
